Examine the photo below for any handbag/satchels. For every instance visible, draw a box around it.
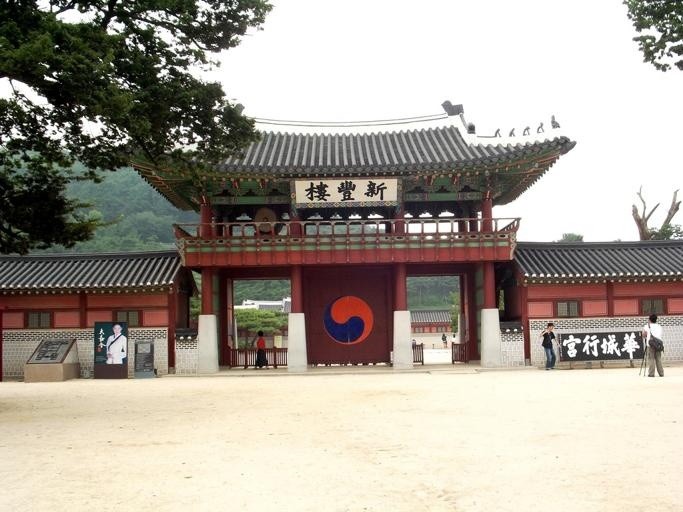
[649,335,664,351]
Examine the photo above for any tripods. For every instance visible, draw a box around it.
[639,346,648,377]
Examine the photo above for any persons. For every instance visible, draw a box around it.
[642,314,664,377]
[106,324,127,364]
[255,331,268,369]
[539,323,561,370]
